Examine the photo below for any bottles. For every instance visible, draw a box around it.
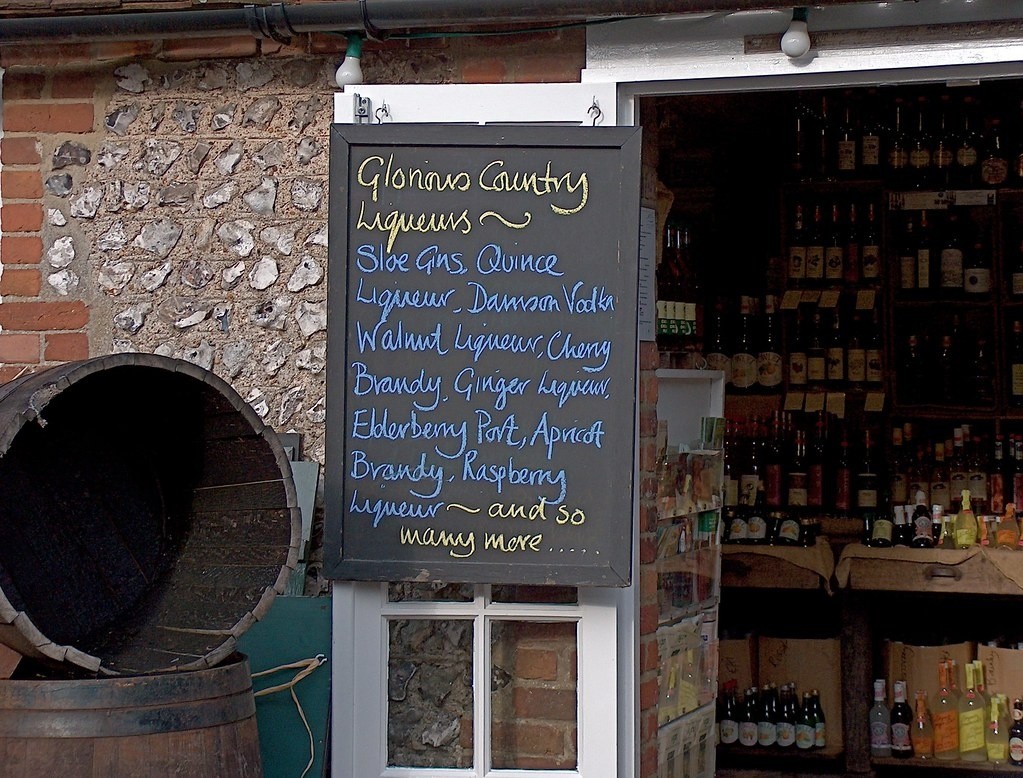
[982,631,1023,651]
[871,658,1023,767]
[720,680,827,752]
[640,86,1023,555]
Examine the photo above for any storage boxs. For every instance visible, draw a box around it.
[850,546,1023,595]
[717,630,757,692]
[884,637,975,711]
[977,641,1023,704]
[720,536,826,588]
[758,636,843,759]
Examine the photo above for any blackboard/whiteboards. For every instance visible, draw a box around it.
[318,118,645,590]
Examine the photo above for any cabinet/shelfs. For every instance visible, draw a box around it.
[655,369,727,778]
[777,177,1023,513]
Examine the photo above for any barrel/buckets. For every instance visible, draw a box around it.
[0,659,260,778]
